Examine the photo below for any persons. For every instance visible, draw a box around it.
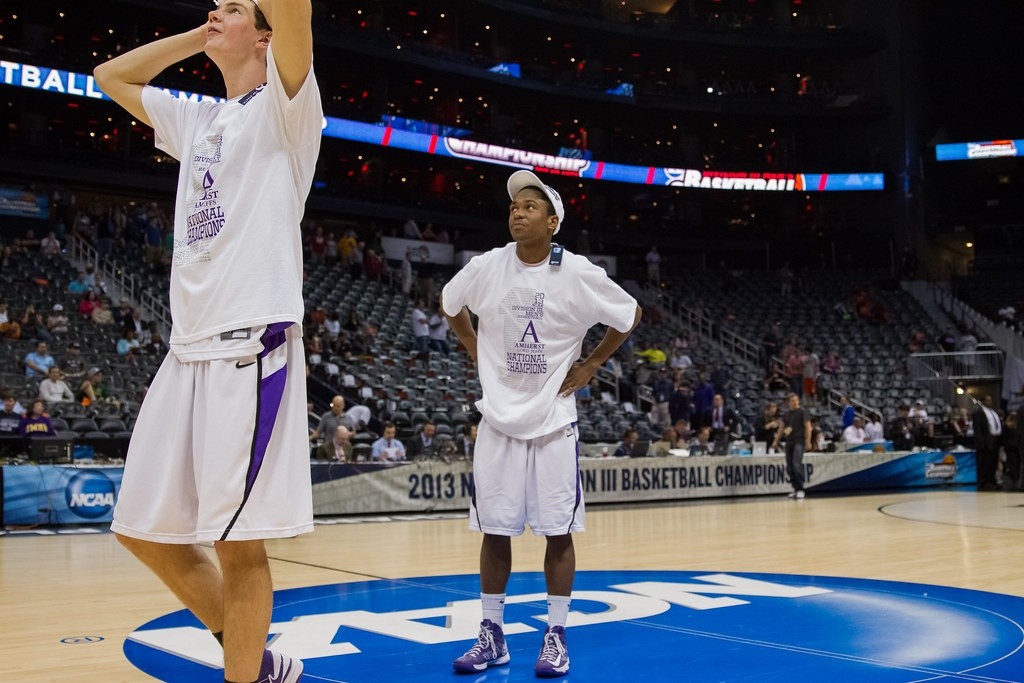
[772,393,812,499]
[94,0,323,683]
[0,199,175,437]
[1003,405,1024,491]
[973,394,1002,492]
[574,248,1024,442]
[441,169,643,677]
[299,223,477,459]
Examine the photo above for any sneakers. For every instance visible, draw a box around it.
[535,624,570,678]
[256,649,304,683]
[452,618,510,675]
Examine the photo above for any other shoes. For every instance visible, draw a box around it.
[788,491,805,500]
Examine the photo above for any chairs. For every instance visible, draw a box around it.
[0,235,952,461]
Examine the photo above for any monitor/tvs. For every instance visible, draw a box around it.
[29,435,73,459]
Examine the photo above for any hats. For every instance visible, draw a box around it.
[507,169,565,234]
[915,400,924,405]
[87,368,102,378]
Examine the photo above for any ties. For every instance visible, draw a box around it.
[388,441,390,448]
[338,446,343,462]
[715,406,719,423]
[990,408,999,432]
[426,438,429,443]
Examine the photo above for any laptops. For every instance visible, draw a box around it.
[752,441,767,455]
[653,441,671,456]
[710,442,729,456]
[630,442,649,457]
[351,447,373,462]
[689,445,704,458]
